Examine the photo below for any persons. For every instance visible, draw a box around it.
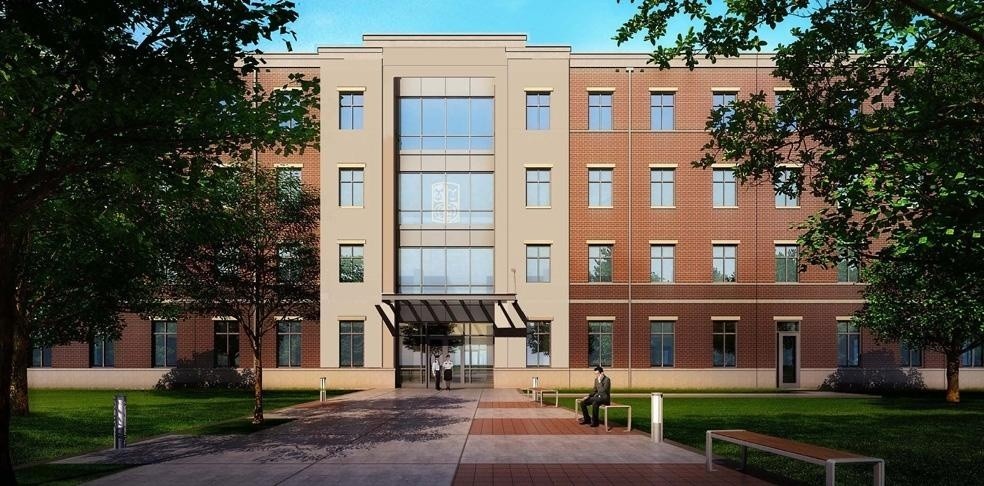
[432,354,441,390]
[579,366,610,428]
[442,355,453,389]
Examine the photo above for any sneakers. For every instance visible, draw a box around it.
[579,420,599,427]
